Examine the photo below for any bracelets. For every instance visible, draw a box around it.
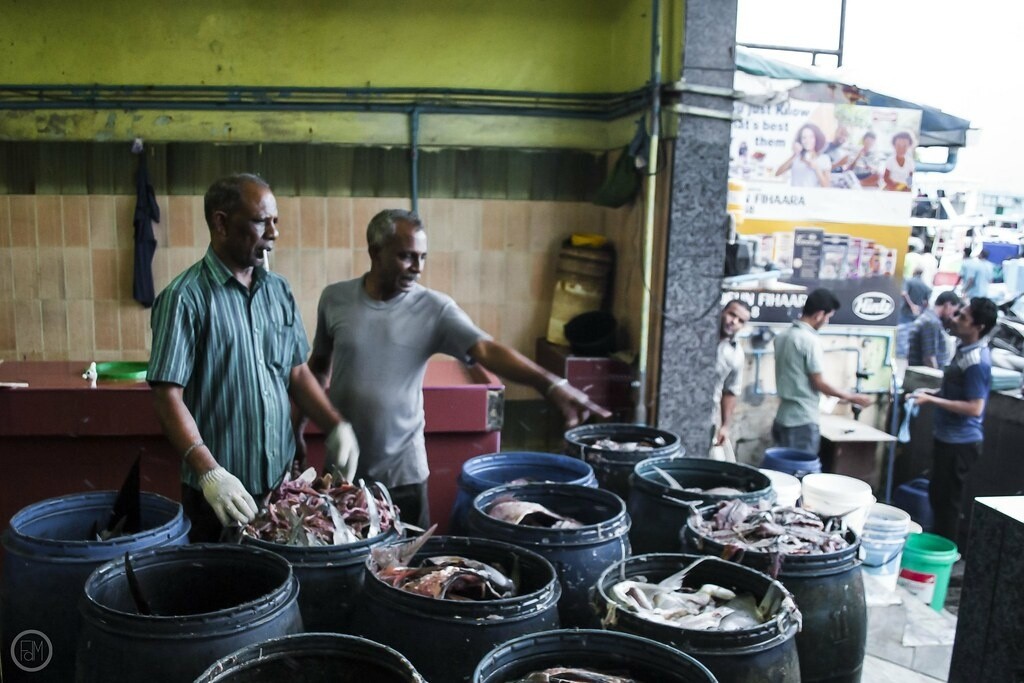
[184,441,205,461]
[546,378,569,395]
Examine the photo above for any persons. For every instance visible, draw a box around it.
[147,172,361,546]
[953,247,994,302]
[910,291,961,368]
[708,299,751,462]
[883,132,916,191]
[772,288,873,456]
[824,124,881,187]
[900,229,938,322]
[293,208,610,536]
[774,123,832,188]
[906,296,998,543]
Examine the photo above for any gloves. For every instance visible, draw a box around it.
[325,422,359,484]
[200,466,258,526]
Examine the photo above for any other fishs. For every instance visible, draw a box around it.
[239,457,425,546]
[369,522,516,600]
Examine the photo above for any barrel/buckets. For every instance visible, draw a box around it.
[76,540,309,682]
[448,451,600,547]
[193,631,431,683]
[228,496,407,631]
[624,456,778,560]
[467,483,633,631]
[677,501,867,682]
[754,446,961,615]
[591,550,808,683]
[563,421,685,536]
[360,535,563,682]
[0,489,196,682]
[471,627,721,683]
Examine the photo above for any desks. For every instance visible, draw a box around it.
[820,412,901,444]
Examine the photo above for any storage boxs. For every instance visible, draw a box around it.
[535,335,634,418]
